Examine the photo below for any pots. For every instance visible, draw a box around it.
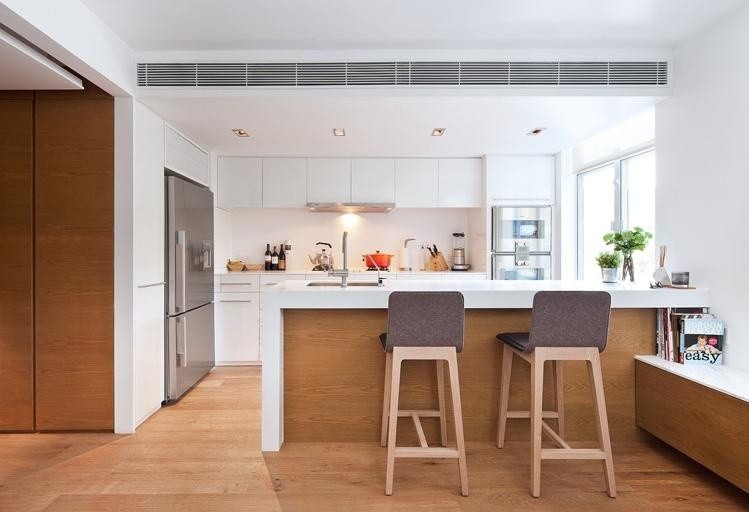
[362,250,394,270]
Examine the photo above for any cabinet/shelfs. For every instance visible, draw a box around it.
[486,154,555,202]
[306,157,351,204]
[352,158,395,202]
[439,159,481,208]
[213,276,307,367]
[395,158,438,208]
[261,158,306,209]
[216,157,260,209]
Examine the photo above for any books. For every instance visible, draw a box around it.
[655,308,725,369]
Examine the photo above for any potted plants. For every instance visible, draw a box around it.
[603,227,653,282]
[597,254,621,283]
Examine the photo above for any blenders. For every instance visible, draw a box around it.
[451,232,470,270]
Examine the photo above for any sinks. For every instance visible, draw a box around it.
[306,281,380,287]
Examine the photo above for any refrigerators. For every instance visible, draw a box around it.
[164,175,215,400]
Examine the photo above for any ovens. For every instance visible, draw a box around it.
[491,206,553,280]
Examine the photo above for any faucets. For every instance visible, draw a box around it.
[341,231,349,270]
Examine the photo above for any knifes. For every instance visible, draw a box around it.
[426,245,438,257]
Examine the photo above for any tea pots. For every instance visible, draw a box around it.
[308,242,334,265]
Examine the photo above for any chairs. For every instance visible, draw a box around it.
[380,291,468,496]
[495,291,620,499]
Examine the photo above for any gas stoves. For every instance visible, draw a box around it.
[312,266,390,272]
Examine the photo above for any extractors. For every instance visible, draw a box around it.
[308,202,394,217]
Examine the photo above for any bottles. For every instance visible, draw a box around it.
[271,246,278,269]
[278,244,285,270]
[264,244,271,270]
